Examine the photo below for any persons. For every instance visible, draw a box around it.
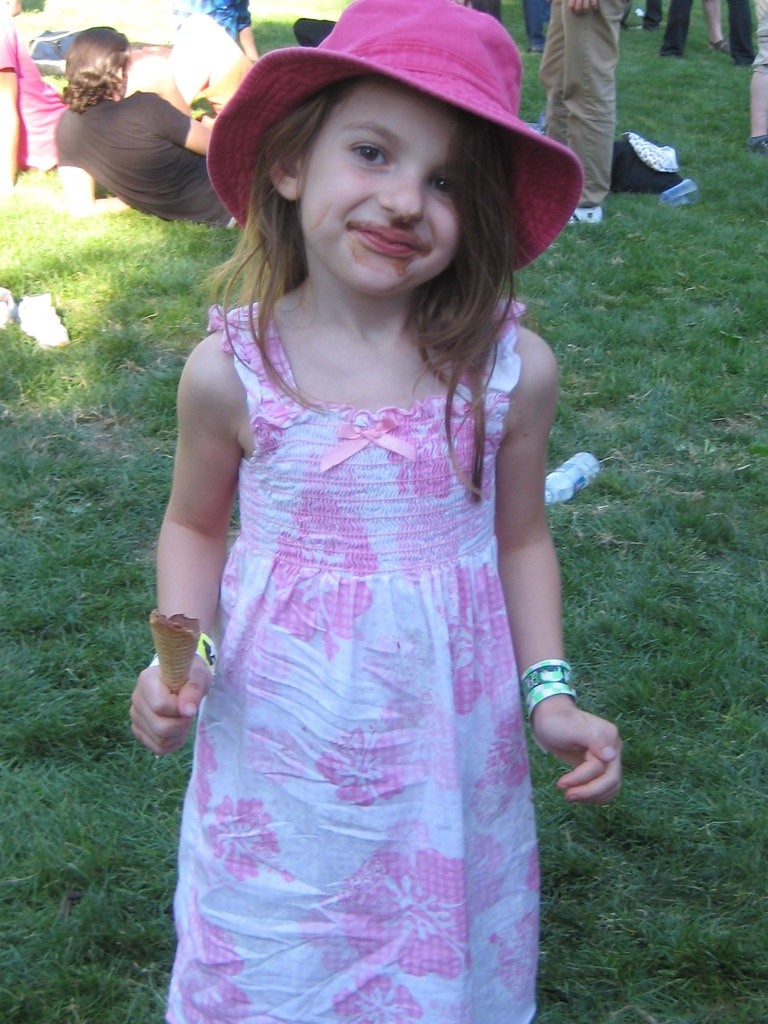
[130,0,622,1024]
[0,0,261,229]
[463,0,768,225]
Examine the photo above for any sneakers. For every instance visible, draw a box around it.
[567,205,602,223]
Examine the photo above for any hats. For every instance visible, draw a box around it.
[207,0,584,272]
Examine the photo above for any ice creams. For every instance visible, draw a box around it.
[148,609,202,698]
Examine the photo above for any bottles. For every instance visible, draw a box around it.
[536,112,547,135]
[544,452,601,507]
[660,179,700,206]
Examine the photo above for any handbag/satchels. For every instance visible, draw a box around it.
[26,30,83,75]
[611,132,683,193]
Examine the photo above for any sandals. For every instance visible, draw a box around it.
[709,37,731,53]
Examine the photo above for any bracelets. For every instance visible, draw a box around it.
[518,659,577,727]
[149,630,218,676]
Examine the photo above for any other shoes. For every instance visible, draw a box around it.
[630,25,645,31]
[528,42,544,53]
[747,134,768,152]
[0,287,15,328]
[18,293,71,349]
